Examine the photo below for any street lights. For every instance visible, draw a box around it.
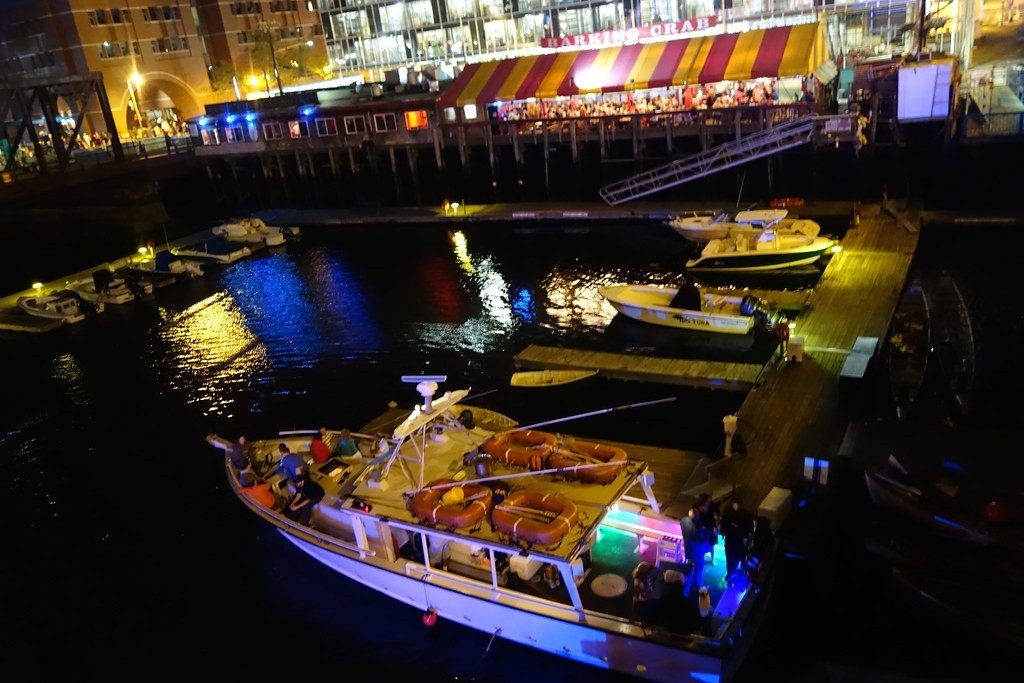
[127,73,148,132]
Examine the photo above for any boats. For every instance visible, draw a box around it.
[207,372,792,683]
[685,230,833,271]
[662,207,822,245]
[858,265,1024,664]
[598,286,785,336]
[15,215,303,324]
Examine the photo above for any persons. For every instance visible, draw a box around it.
[333,429,363,466]
[470,495,779,614]
[286,475,325,528]
[670,274,701,312]
[370,432,391,464]
[489,81,838,141]
[231,434,264,476]
[236,458,267,487]
[0,112,191,172]
[427,532,454,573]
[275,428,336,494]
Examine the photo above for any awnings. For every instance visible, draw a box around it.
[437,19,838,113]
